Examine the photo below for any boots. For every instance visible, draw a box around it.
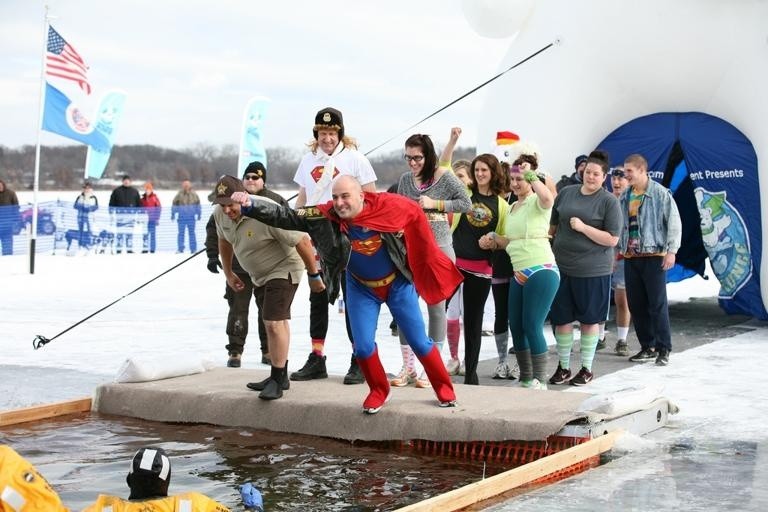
[344,354,365,384]
[355,342,392,414]
[291,352,329,380]
[417,343,458,407]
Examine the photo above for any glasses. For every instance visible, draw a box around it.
[404,153,426,162]
[245,175,261,180]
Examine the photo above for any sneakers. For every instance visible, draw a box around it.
[390,366,432,388]
[117,249,155,253]
[175,251,183,254]
[616,339,628,356]
[655,348,669,366]
[445,358,466,376]
[629,349,658,362]
[549,361,572,385]
[492,360,548,389]
[596,337,607,351]
[569,368,594,386]
[226,352,271,367]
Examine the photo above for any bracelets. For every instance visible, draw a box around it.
[306,271,320,281]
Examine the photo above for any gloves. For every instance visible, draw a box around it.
[237,483,265,510]
[207,257,222,274]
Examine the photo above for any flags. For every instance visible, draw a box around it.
[43,22,92,97]
[37,78,113,156]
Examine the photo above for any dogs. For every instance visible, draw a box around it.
[65,229,102,250]
[95,230,114,254]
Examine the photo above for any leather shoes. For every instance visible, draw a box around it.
[247,375,290,399]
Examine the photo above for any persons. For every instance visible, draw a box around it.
[206,161,290,368]
[290,106,379,383]
[207,174,328,401]
[72,178,99,258]
[0,179,22,256]
[76,446,264,512]
[108,174,140,254]
[138,182,162,253]
[596,153,684,365]
[385,128,624,389]
[170,180,202,253]
[230,173,465,414]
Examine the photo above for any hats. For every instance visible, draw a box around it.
[130,446,171,486]
[144,184,152,189]
[243,161,266,183]
[211,175,245,205]
[575,155,588,168]
[123,175,130,180]
[313,107,344,141]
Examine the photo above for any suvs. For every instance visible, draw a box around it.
[11,202,55,234]
